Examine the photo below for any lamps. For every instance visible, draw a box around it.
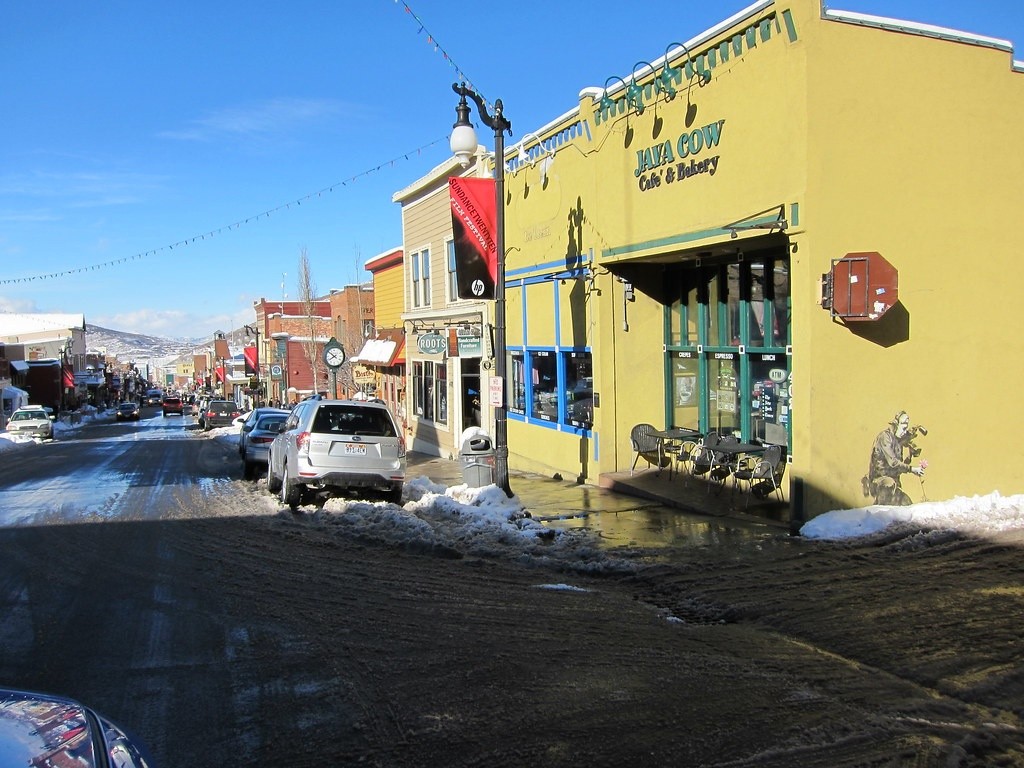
[600,76,645,115]
[375,325,385,339]
[628,61,676,102]
[661,43,711,85]
[483,155,518,178]
[400,319,418,334]
[364,324,375,337]
[412,319,434,334]
[518,133,555,162]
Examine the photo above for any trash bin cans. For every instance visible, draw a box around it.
[458,427,494,487]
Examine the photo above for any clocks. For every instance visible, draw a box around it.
[325,346,345,368]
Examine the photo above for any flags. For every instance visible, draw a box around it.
[192,378,203,391]
[206,377,211,387]
[244,348,258,377]
[216,368,223,382]
[449,175,496,300]
[63,365,74,388]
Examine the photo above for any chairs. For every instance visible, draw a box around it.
[627,424,790,512]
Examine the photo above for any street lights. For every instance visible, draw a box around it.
[59,344,67,412]
[243,324,261,408]
[448,79,516,494]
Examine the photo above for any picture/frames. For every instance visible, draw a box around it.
[674,374,698,408]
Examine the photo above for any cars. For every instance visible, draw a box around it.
[141,384,224,422]
[242,413,291,477]
[5,405,56,440]
[263,398,405,503]
[117,402,140,421]
[237,407,274,455]
[200,401,241,432]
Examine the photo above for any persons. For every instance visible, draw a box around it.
[269,396,297,410]
